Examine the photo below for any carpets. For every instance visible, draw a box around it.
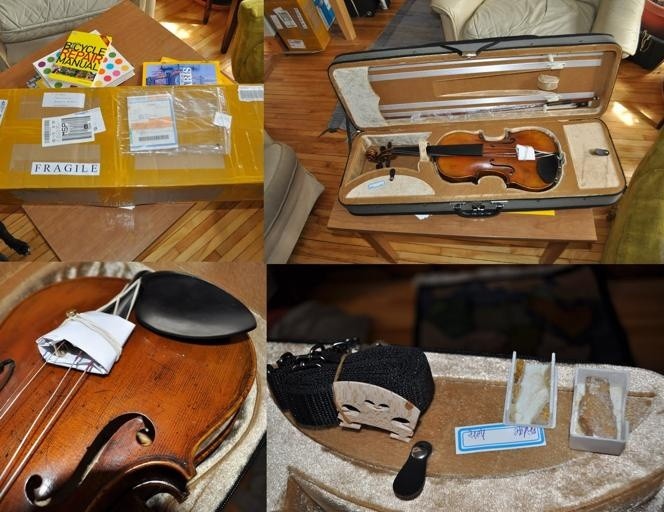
[329,0,449,133]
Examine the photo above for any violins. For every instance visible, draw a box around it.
[0,272,258,512]
[366,130,563,192]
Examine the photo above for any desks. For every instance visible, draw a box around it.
[325,189,598,263]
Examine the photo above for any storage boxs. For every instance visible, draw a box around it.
[264,0,331,50]
[1,84,263,206]
[313,0,337,31]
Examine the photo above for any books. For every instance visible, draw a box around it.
[142,61,222,85]
[47,30,113,87]
[33,29,135,88]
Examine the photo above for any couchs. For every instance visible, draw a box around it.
[434,0,648,67]
[231,0,263,83]
[264,126,327,263]
[0,1,156,74]
